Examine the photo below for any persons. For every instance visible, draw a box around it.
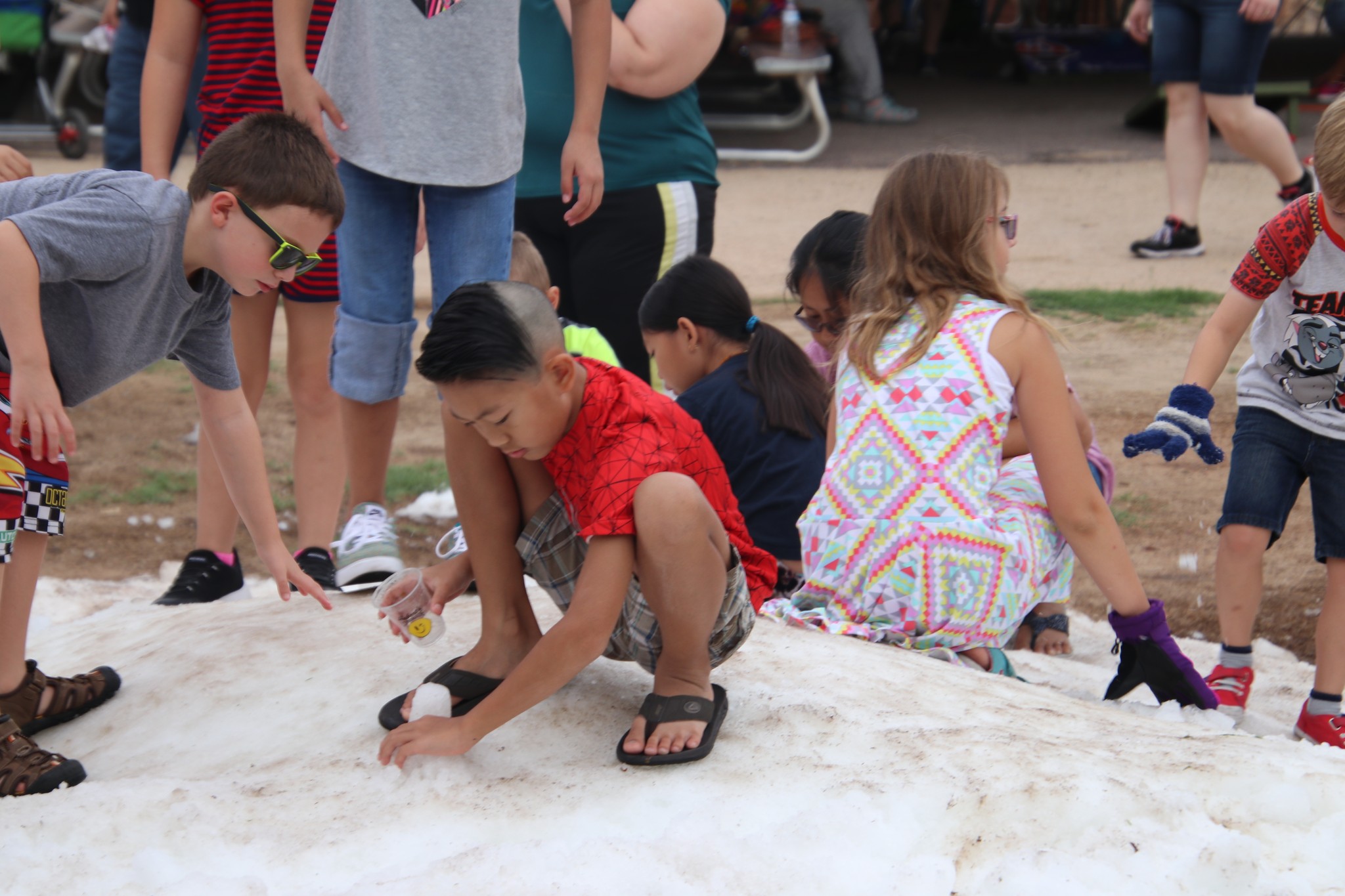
[0,0,1112,709]
[1121,1,1319,263]
[376,279,777,770]
[759,149,1219,710]
[1122,93,1345,747]
[0,110,346,797]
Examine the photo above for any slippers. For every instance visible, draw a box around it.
[617,683,728,766]
[379,655,505,732]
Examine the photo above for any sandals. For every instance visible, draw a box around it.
[0,715,87,797]
[1013,609,1071,659]
[0,659,120,736]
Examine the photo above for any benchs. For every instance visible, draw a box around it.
[735,33,832,76]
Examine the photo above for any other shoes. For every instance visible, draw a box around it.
[835,92,918,125]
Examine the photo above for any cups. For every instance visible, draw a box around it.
[372,568,446,647]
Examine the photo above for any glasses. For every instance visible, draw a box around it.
[984,209,1018,241]
[205,184,323,275]
[792,303,850,336]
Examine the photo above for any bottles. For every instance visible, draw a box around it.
[779,0,801,59]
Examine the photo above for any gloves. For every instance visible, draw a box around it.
[1107,596,1218,712]
[1123,385,1224,466]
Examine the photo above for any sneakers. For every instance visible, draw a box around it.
[1275,166,1320,208]
[1202,664,1253,724]
[1293,697,1345,749]
[435,524,479,593]
[330,502,403,586]
[288,547,343,599]
[1129,218,1205,258]
[152,547,245,607]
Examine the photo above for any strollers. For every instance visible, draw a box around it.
[33,0,121,161]
